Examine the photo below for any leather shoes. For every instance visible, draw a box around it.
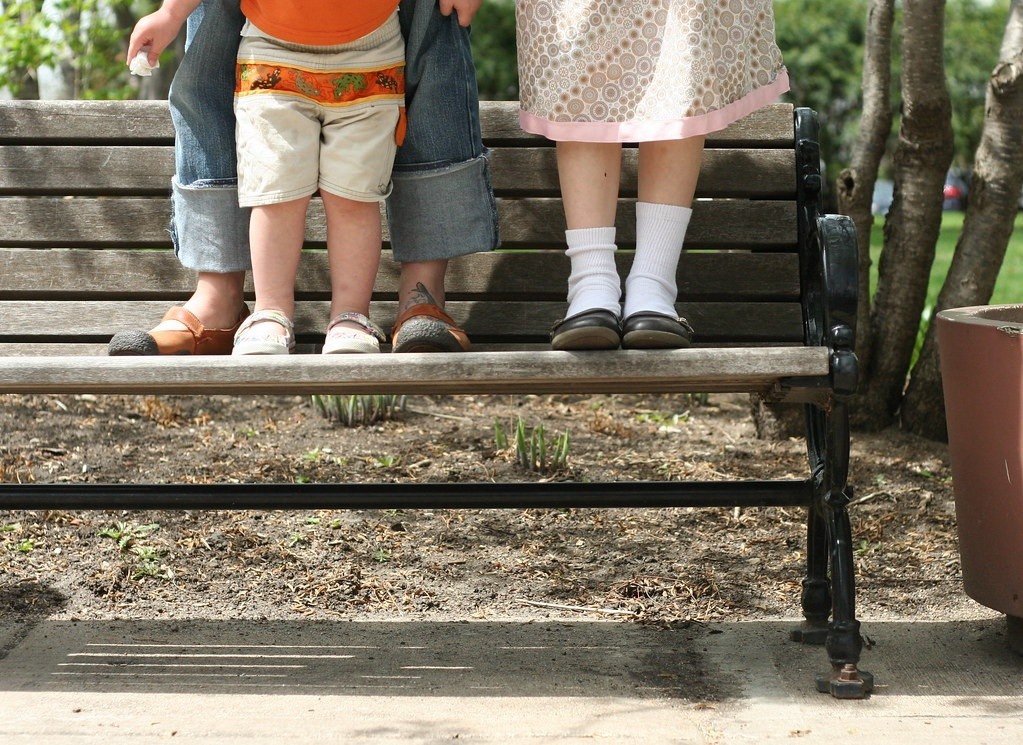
[548,309,620,349]
[621,316,694,348]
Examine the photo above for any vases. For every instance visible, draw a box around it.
[935,302,1023,654]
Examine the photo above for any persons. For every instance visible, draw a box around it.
[107,0,498,354]
[548,0,706,349]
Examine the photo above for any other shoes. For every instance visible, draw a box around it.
[322,312,387,354]
[232,310,296,354]
[391,306,472,353]
[108,305,250,356]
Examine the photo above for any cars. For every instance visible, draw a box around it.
[873,168,970,215]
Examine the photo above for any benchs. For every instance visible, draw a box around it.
[2,101,879,704]
